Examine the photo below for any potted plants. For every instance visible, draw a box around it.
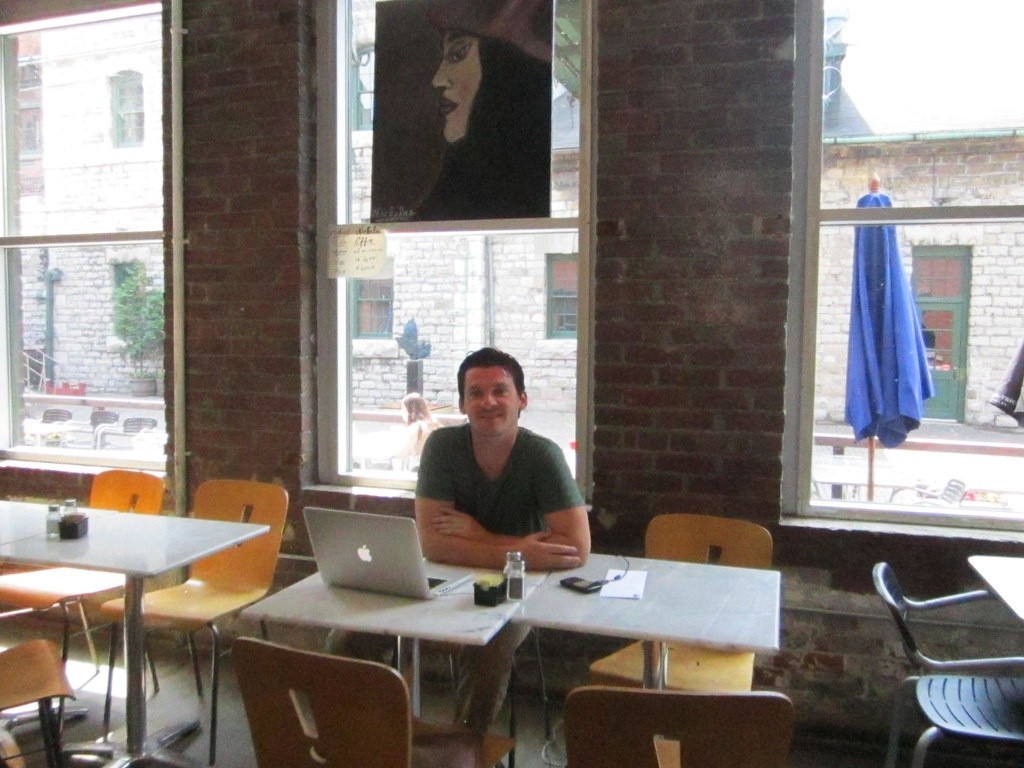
[111,259,166,399]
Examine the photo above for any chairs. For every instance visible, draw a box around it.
[101,478,290,765]
[21,407,158,455]
[229,633,514,767]
[0,472,163,681]
[554,681,796,768]
[1,640,81,768]
[584,511,773,695]
[869,560,1023,768]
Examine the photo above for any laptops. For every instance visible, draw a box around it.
[302,506,474,600]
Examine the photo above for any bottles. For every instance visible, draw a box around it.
[63,499,78,518]
[46,504,63,537]
[506,560,527,601]
[502,552,522,582]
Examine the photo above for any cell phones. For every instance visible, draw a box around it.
[560,577,602,593]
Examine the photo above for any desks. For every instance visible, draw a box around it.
[0,497,271,768]
[239,539,553,720]
[515,550,783,692]
[965,554,1023,623]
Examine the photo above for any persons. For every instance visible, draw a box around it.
[412,346,592,570]
[394,392,444,476]
